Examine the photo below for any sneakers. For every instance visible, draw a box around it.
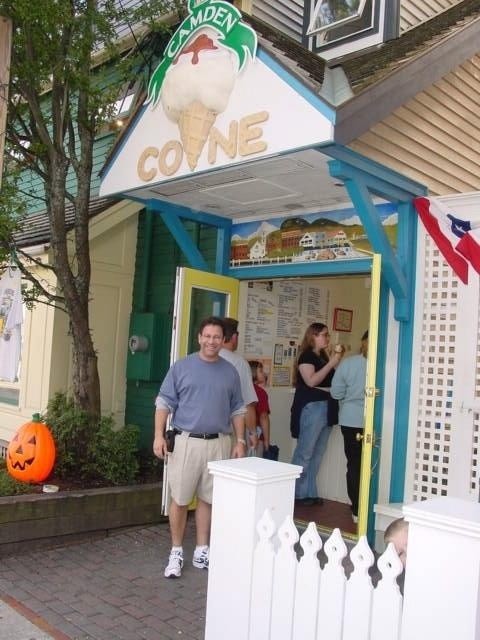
[164,550,184,578]
[296,497,323,505]
[352,512,358,524]
[192,546,208,569]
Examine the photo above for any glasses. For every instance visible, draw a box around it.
[315,332,329,337]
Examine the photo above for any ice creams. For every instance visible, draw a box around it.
[161,33,235,172]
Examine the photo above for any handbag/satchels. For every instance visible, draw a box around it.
[264,444,279,461]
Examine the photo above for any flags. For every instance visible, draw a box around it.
[413,195,480,286]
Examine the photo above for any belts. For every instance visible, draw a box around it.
[176,430,219,439]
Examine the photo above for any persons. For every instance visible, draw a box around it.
[291,323,338,505]
[153,316,270,578]
[330,329,368,524]
[384,517,409,595]
